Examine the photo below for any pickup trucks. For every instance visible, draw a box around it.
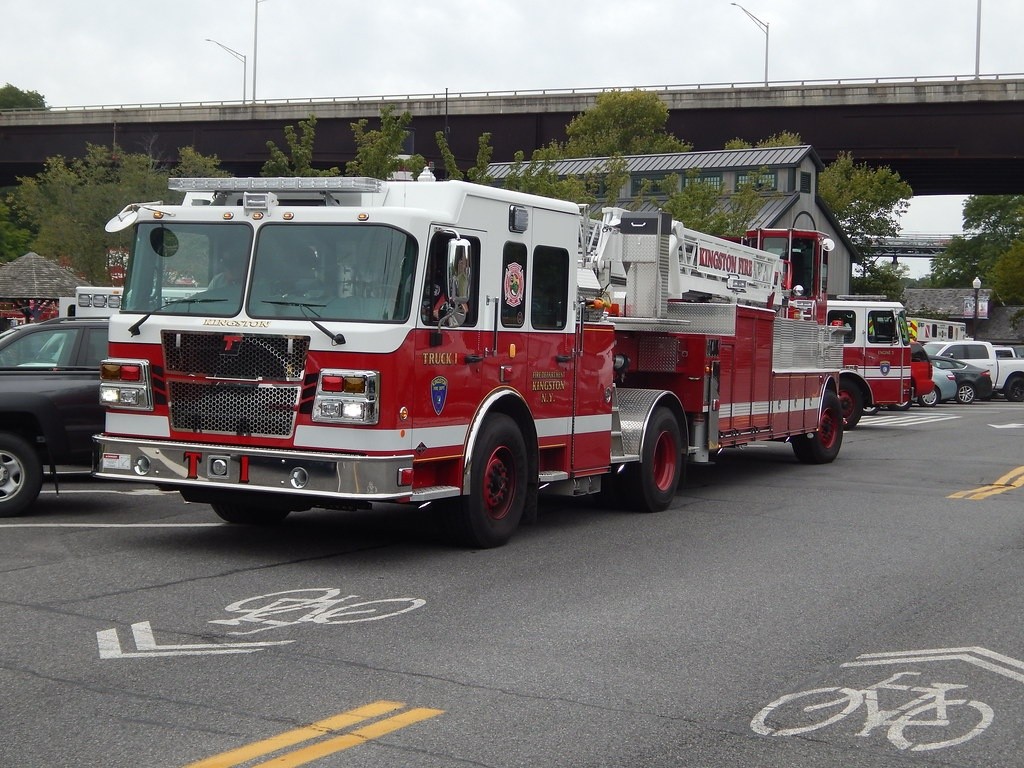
[922,341,1024,401]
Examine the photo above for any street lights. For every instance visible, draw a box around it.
[862,248,899,280]
[730,3,769,88]
[206,39,246,104]
[972,277,981,341]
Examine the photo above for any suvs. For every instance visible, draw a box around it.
[889,343,934,410]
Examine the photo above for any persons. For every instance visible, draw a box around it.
[205,249,246,290]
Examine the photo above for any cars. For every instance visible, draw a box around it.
[1,315,108,517]
[917,367,957,408]
[1013,346,1024,358]
[993,345,1016,359]
[928,355,994,405]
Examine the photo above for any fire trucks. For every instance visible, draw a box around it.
[825,296,912,428]
[87,175,851,549]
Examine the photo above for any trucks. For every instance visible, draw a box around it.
[15,283,210,368]
[905,317,966,345]
